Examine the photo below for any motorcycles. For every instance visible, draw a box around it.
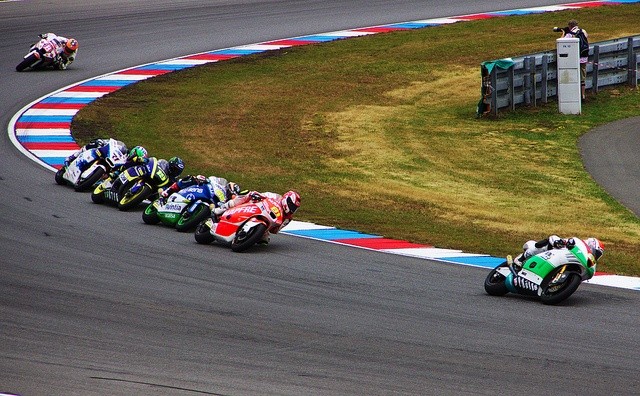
[16,34,67,71]
[194,191,283,252]
[92,157,170,211]
[485,237,597,305]
[55,143,129,192]
[142,177,226,232]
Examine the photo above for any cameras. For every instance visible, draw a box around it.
[552,26,569,33]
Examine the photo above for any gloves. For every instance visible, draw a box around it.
[249,190,258,199]
[56,57,62,65]
[38,32,46,39]
[94,139,105,147]
[553,239,564,249]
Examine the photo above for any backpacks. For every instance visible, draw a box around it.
[568,28,589,57]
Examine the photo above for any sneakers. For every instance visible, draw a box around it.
[513,253,524,269]
[161,188,171,197]
[66,153,78,161]
[212,204,226,216]
[109,169,119,179]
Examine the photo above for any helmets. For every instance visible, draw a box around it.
[281,190,301,216]
[66,38,78,53]
[585,237,605,262]
[130,146,148,163]
[168,156,185,177]
[229,182,240,196]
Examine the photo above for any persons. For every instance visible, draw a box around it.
[559,20,590,100]
[157,157,184,182]
[116,146,147,169]
[160,175,241,199]
[514,234,604,277]
[212,190,301,247]
[56,35,79,70]
[67,138,127,161]
